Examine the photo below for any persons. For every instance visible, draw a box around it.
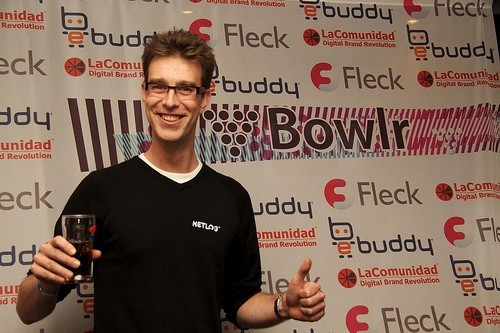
[16,27,326,333]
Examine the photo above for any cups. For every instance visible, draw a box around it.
[61,215,95,281]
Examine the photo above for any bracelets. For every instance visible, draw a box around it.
[273,292,291,321]
[35,275,60,297]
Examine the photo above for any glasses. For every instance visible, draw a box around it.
[145,80,206,99]
